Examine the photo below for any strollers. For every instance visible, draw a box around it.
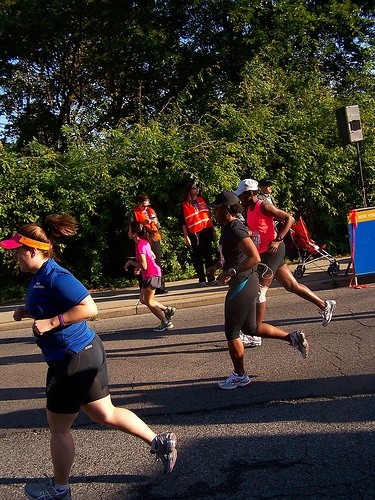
[280,216,340,280]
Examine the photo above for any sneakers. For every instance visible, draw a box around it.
[239,333,262,346]
[208,280,218,286]
[290,330,309,359]
[199,282,208,288]
[154,320,174,331]
[25,483,72,500]
[156,433,178,475]
[165,306,176,322]
[218,372,251,389]
[319,300,336,327]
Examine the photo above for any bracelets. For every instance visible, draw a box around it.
[58,313,65,326]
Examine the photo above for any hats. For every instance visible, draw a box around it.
[259,179,273,185]
[0,233,53,250]
[234,179,259,196]
[207,192,239,207]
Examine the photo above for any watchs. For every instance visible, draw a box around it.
[229,268,237,276]
[276,237,283,240]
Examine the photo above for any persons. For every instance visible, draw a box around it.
[235,179,336,347]
[259,179,276,208]
[207,243,225,273]
[207,192,309,388]
[125,222,175,330]
[0,214,177,500]
[131,194,168,293]
[179,184,216,287]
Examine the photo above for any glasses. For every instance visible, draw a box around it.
[139,204,151,207]
[191,186,200,189]
